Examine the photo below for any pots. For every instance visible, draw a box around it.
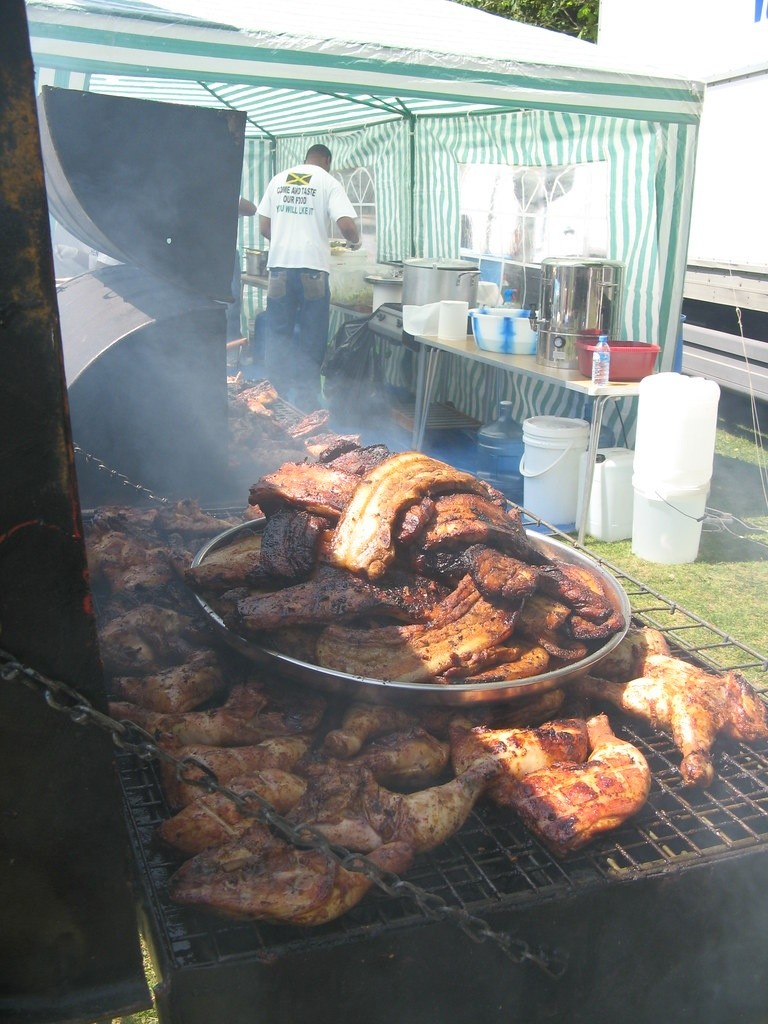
[403,258,481,309]
[242,251,269,276]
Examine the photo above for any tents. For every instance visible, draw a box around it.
[27,1,704,453]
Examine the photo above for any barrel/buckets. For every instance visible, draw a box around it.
[332,250,368,263]
[518,416,589,524]
[632,371,721,565]
[372,284,403,317]
[576,448,632,543]
[529,257,625,370]
[437,301,469,341]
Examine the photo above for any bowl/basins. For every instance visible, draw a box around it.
[465,304,540,354]
[574,336,661,382]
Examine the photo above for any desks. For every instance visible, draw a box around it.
[236,269,374,318]
[411,334,642,545]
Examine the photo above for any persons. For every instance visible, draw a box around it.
[256,144,363,412]
[217,197,257,367]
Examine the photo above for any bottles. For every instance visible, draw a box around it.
[592,336,611,386]
[475,401,522,499]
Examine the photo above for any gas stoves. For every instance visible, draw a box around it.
[367,305,412,344]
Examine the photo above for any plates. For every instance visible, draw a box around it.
[365,276,403,285]
[189,516,631,707]
[329,239,347,247]
